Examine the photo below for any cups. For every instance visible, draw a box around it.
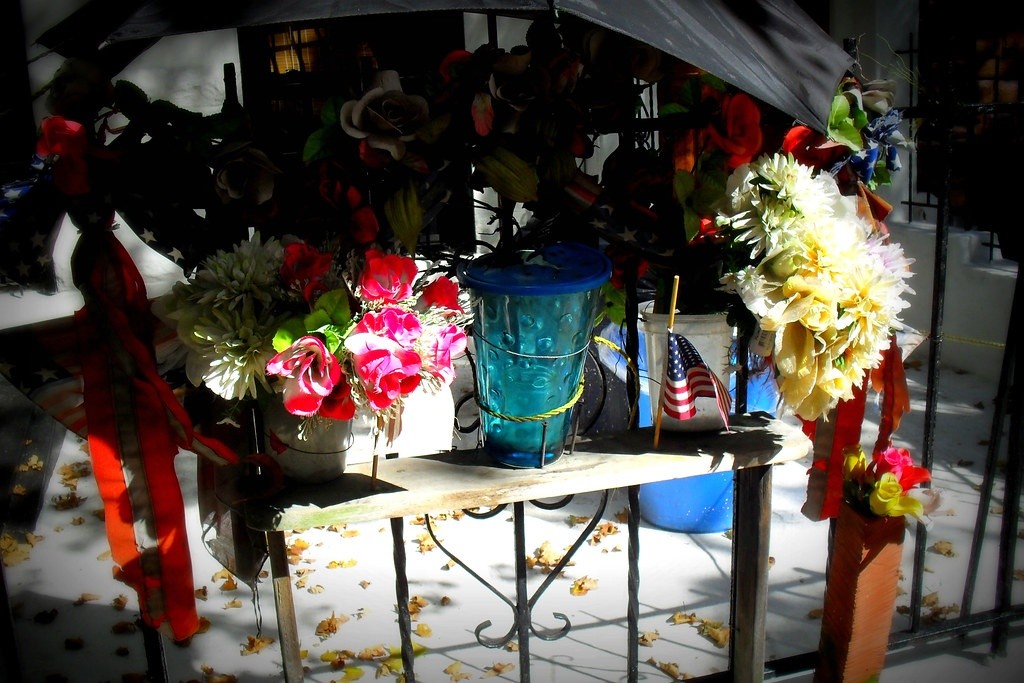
[463,244,613,467]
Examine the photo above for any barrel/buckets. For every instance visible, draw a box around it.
[615,327,778,535]
[640,307,733,432]
[251,382,355,482]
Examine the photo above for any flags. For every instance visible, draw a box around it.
[663,331,733,432]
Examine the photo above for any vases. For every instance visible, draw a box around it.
[262,403,356,485]
[810,498,908,683]
[639,301,734,432]
[461,249,614,467]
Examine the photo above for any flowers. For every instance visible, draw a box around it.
[31,18,912,330]
[149,236,474,456]
[715,152,917,429]
[839,442,942,534]
